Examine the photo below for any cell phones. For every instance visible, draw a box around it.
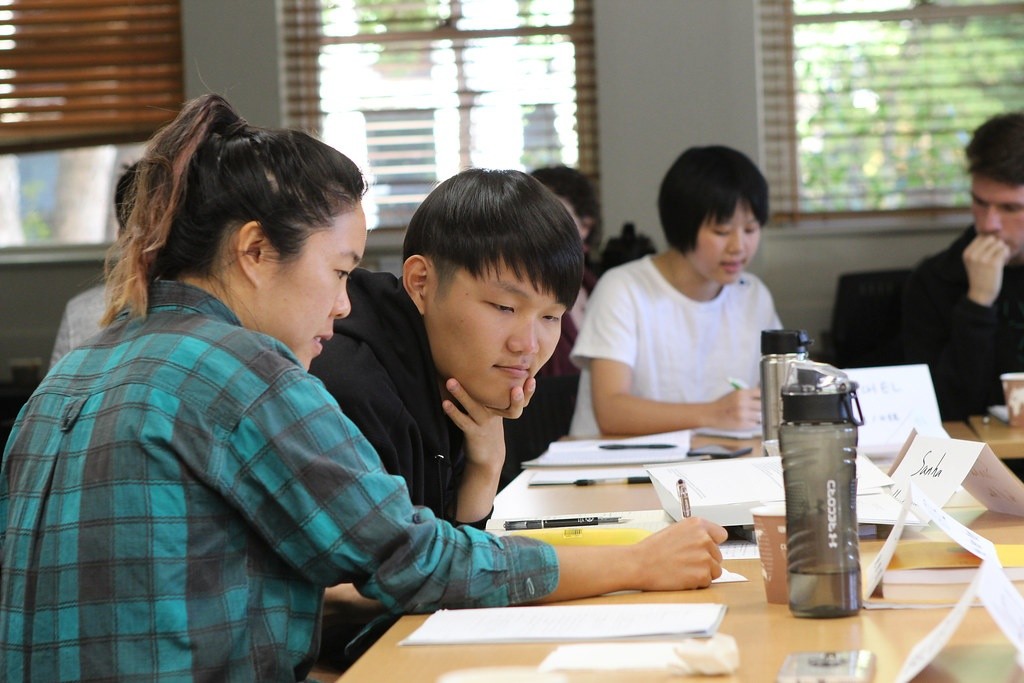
[687,444,752,459]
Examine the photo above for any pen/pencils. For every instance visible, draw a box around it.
[574,476,652,486]
[504,516,631,529]
[727,376,743,390]
[677,479,691,519]
[599,444,678,449]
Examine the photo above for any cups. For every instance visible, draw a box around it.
[752,507,788,605]
[1000,373,1024,426]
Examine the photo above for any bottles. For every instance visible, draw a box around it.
[761,328,814,456]
[777,360,865,618]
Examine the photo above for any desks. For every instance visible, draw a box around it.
[332,416,1024,683]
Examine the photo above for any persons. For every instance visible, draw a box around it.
[568,144,784,447]
[856,112,1024,433]
[310,168,585,670]
[499,162,596,485]
[0,93,727,683]
[46,161,142,373]
